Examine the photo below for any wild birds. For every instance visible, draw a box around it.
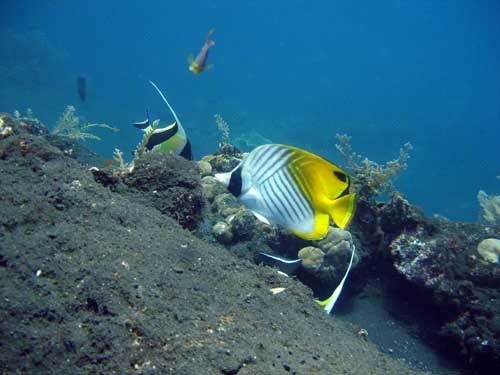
[313,245,356,315]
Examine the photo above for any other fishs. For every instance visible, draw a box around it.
[214,143,358,242]
[132,80,195,162]
[186,27,218,74]
